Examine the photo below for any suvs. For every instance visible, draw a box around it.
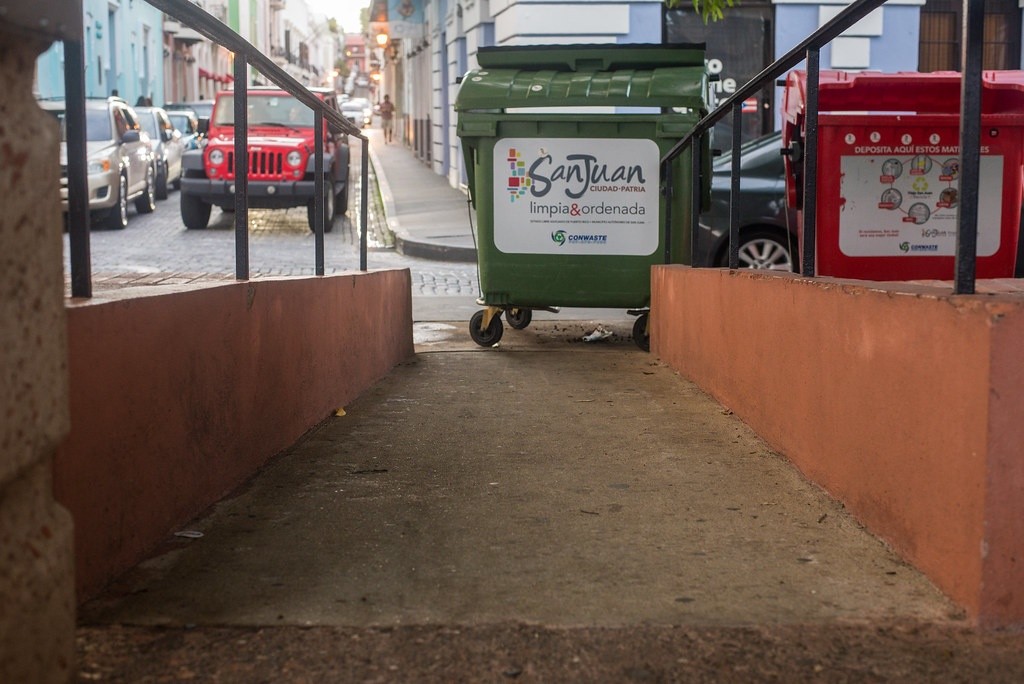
[36,96,157,229]
[179,87,349,234]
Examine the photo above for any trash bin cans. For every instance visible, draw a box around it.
[773,68,1024,282]
[453,40,713,351]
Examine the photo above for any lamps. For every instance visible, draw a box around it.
[377,29,387,44]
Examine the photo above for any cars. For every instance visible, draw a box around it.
[336,93,373,129]
[164,103,215,150]
[132,106,185,200]
[697,129,799,271]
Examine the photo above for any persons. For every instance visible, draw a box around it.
[379,95,394,144]
[288,105,304,123]
[111,89,118,98]
[134,96,153,107]
